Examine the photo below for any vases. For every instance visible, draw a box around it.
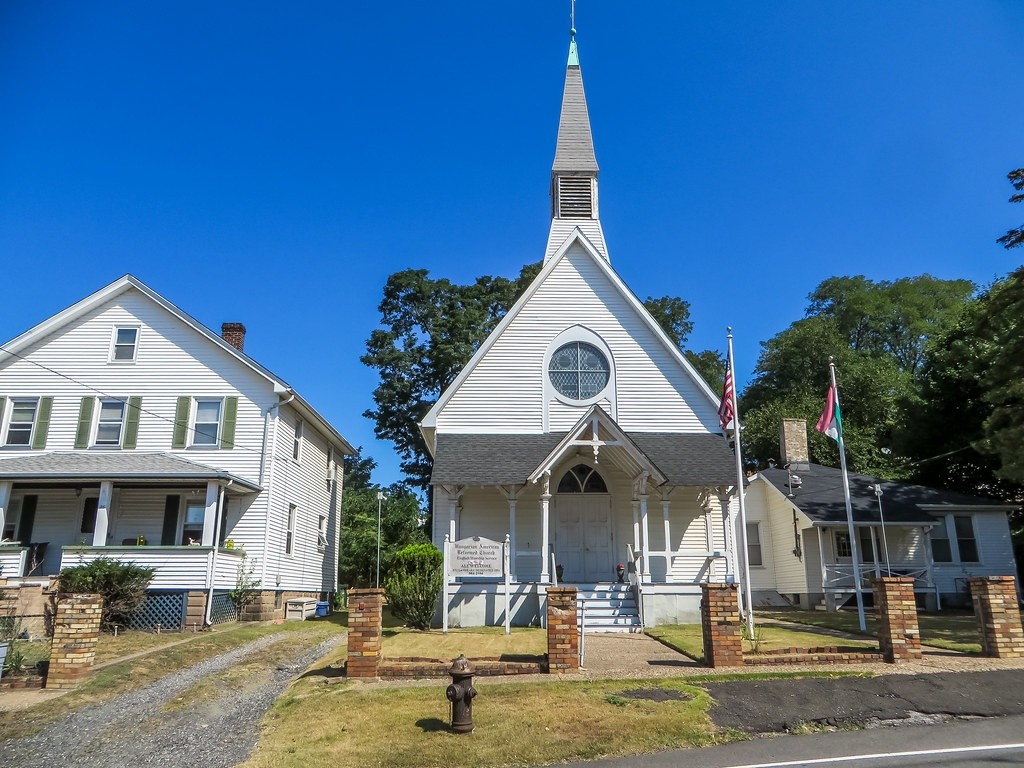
[0,643,9,683]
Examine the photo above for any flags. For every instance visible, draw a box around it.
[816,373,845,450]
[718,343,735,432]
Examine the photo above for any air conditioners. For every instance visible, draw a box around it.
[326,469,337,483]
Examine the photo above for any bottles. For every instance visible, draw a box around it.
[139,535,145,546]
[227,540,234,549]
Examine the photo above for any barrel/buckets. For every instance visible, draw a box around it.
[316,601,329,616]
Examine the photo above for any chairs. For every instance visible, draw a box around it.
[23,542,50,578]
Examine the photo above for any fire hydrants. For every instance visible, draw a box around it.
[447,654,480,734]
